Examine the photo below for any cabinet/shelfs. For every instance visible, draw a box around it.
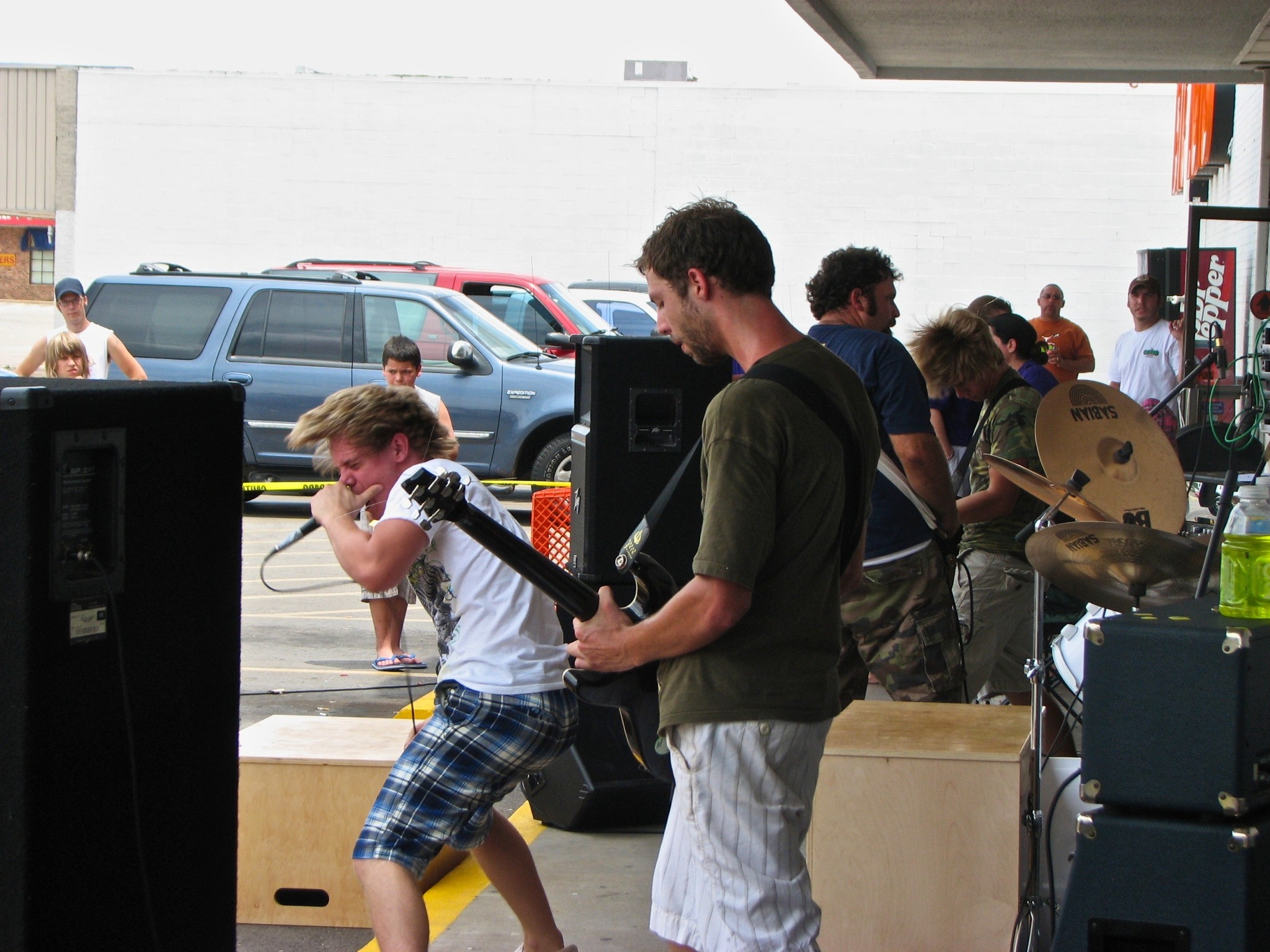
[797,698,1047,952]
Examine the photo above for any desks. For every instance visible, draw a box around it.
[235,714,430,928]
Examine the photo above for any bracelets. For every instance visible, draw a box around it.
[948,451,955,460]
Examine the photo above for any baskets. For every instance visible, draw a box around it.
[531,488,572,612]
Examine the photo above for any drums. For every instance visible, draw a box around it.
[1050,601,1124,702]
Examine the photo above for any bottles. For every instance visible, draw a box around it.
[1219,477,1270,619]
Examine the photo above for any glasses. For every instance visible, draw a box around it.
[57,296,84,306]
[1041,293,1063,301]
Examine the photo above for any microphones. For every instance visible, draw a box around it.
[269,518,321,556]
[1214,323,1227,379]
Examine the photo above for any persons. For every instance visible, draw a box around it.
[1106,271,1186,437]
[1027,284,1095,386]
[45,331,91,380]
[354,336,459,671]
[806,246,970,703]
[15,276,150,381]
[281,386,585,952]
[907,306,1077,757]
[929,294,1012,500]
[567,192,882,952]
[987,313,1060,397]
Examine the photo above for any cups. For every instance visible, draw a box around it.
[1048,346,1059,362]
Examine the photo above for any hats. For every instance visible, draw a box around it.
[1129,274,1161,294]
[55,278,84,299]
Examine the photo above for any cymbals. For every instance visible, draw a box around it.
[981,451,1121,524]
[1035,380,1188,536]
[1025,520,1223,613]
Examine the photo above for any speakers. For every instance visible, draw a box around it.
[1047,593,1270,952]
[518,701,675,833]
[0,372,233,952]
[564,334,735,589]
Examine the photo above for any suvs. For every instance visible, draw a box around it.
[85,258,672,499]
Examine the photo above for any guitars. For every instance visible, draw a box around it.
[400,467,681,783]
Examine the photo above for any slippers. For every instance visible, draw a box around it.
[399,654,427,668]
[513,941,578,952]
[372,655,405,670]
